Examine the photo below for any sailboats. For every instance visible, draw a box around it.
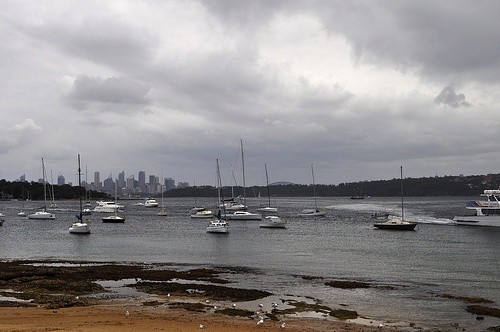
[68,153,92,235]
[221,139,264,220]
[205,172,230,232]
[158,185,169,216]
[189,158,247,218]
[47,169,57,208]
[298,164,335,218]
[28,158,57,220]
[83,166,126,216]
[101,178,127,222]
[257,163,279,212]
[373,166,419,230]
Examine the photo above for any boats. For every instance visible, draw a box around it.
[0,212,6,226]
[135,201,144,206]
[350,196,366,201]
[258,216,288,228]
[145,196,159,208]
[18,209,26,217]
[451,190,500,227]
